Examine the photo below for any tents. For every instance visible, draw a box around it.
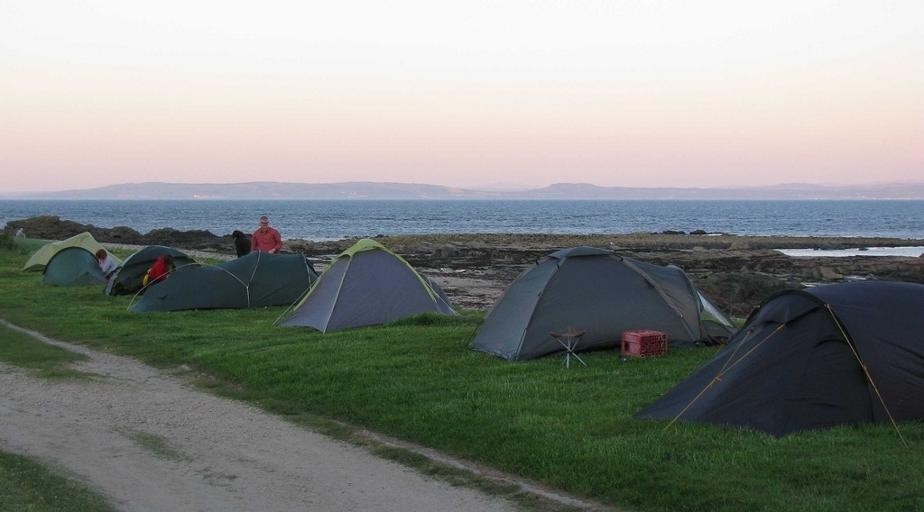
[25,230,319,315]
[465,245,739,363]
[634,281,923,435]
[274,237,461,335]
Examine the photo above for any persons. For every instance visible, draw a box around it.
[250,215,282,253]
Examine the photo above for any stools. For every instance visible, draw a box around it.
[551,330,589,369]
